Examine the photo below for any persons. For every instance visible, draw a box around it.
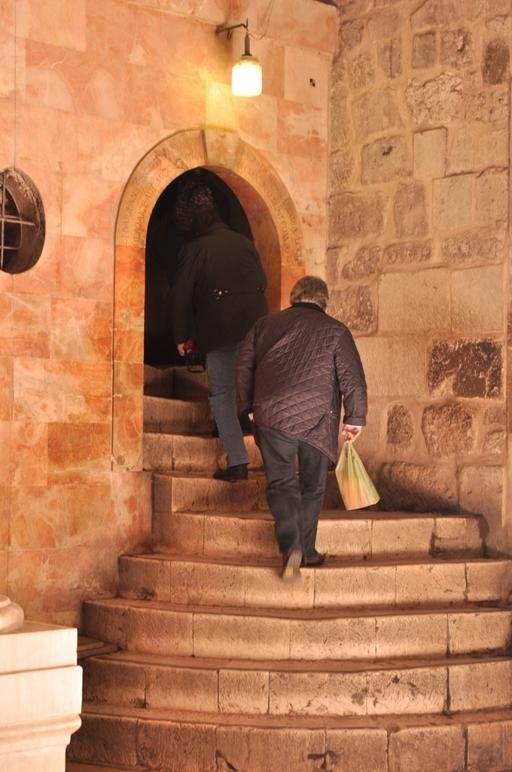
[172,208,270,481]
[236,275,368,582]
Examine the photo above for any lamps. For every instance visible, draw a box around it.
[216,19,263,98]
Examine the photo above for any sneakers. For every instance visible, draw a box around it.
[304,551,325,569]
[213,464,249,481]
[283,550,304,579]
